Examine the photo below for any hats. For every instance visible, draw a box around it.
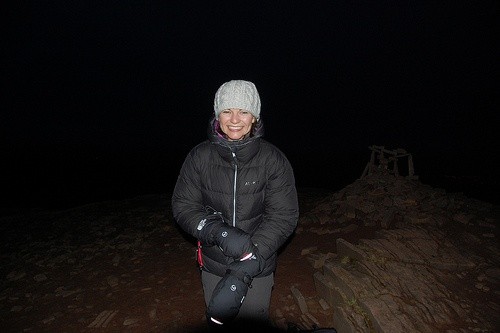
[214,80,261,119]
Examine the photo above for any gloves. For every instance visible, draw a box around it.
[206,261,258,328]
[202,221,251,261]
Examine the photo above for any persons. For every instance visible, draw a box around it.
[172,80,300,325]
